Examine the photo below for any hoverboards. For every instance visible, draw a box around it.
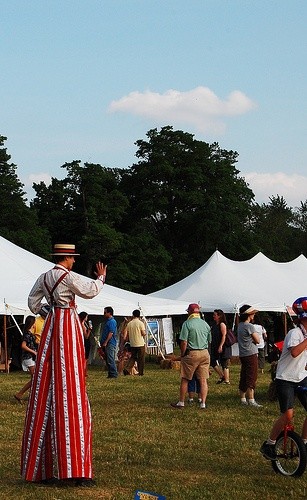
[271,386,307,480]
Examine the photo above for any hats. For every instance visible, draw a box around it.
[49,244,81,257]
[186,304,200,312]
[242,306,258,315]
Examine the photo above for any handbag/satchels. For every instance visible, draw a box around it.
[224,328,237,347]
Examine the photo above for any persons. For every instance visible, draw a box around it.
[259,296,307,462]
[169,303,212,410]
[251,316,268,374]
[32,314,46,353]
[24,242,109,487]
[118,309,148,377]
[210,309,233,385]
[78,311,92,378]
[236,304,263,407]
[99,306,118,379]
[13,315,41,406]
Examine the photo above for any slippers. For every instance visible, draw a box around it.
[14,395,23,405]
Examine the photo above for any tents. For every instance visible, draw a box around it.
[147,250,307,362]
[0,233,198,375]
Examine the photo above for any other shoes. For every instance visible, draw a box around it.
[260,440,277,461]
[197,399,202,403]
[239,400,248,406]
[216,376,226,384]
[199,405,206,409]
[248,402,262,407]
[123,368,129,376]
[169,402,178,408]
[139,372,143,376]
[220,381,231,385]
[187,398,194,404]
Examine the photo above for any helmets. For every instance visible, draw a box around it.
[292,296,307,319]
